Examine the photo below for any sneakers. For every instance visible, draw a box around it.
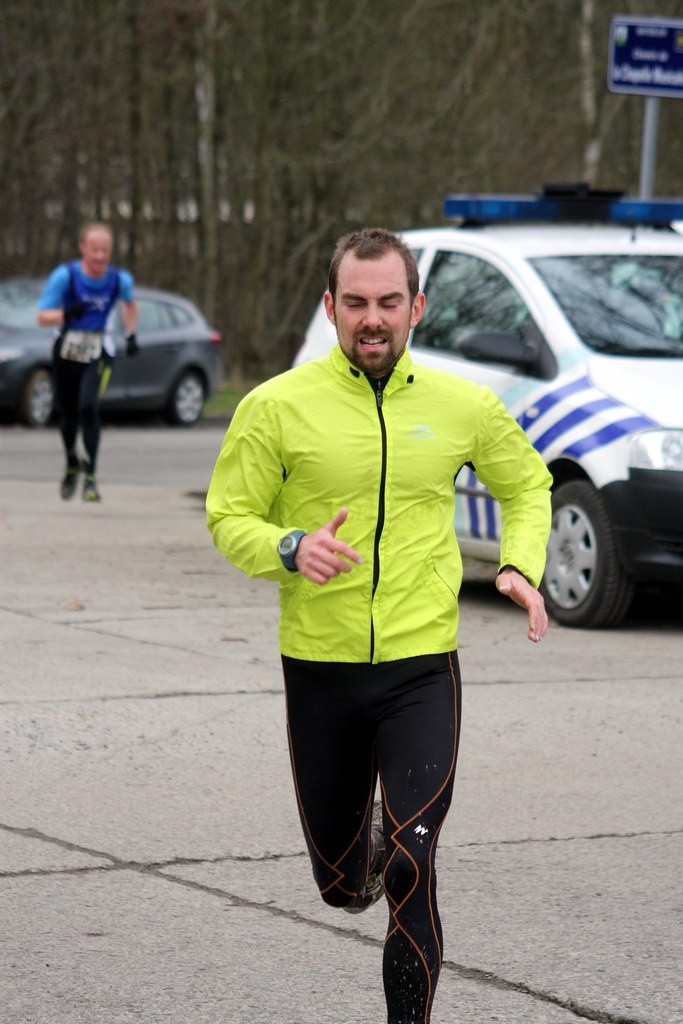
[343,799,386,915]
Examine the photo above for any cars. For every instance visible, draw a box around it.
[287,181,683,632]
[0,275,225,431]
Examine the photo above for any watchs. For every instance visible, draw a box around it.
[278,530,306,572]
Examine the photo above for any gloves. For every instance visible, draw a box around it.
[126,333,138,358]
[64,301,87,322]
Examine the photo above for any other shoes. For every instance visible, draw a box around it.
[81,475,100,502]
[60,466,79,499]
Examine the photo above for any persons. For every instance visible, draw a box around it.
[205,228,555,1023]
[37,222,141,503]
